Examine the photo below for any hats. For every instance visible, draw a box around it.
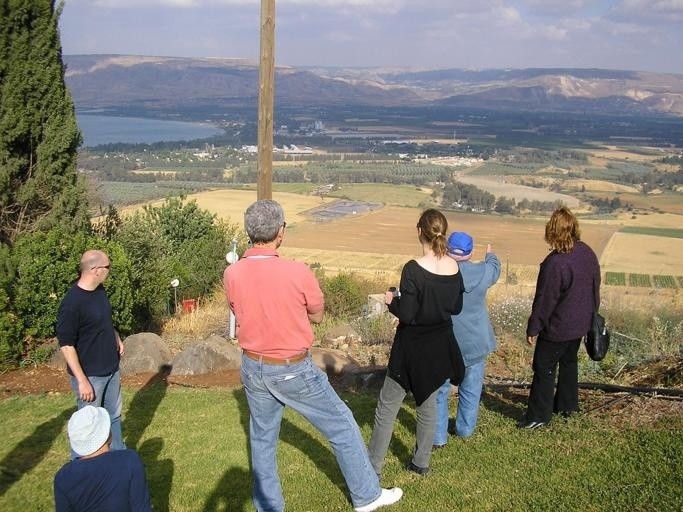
[447,232,473,255]
[67,405,111,456]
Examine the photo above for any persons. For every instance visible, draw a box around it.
[56,250,127,461]
[515,206,601,430]
[367,208,466,480]
[432,232,501,449]
[55,406,152,511]
[222,199,403,512]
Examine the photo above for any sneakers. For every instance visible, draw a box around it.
[409,463,429,474]
[354,487,403,512]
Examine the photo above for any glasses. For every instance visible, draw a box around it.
[92,264,110,270]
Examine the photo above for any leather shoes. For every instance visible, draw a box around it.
[517,420,547,431]
[554,410,570,418]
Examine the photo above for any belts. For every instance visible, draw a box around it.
[241,348,308,365]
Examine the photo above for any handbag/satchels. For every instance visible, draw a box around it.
[585,314,611,361]
